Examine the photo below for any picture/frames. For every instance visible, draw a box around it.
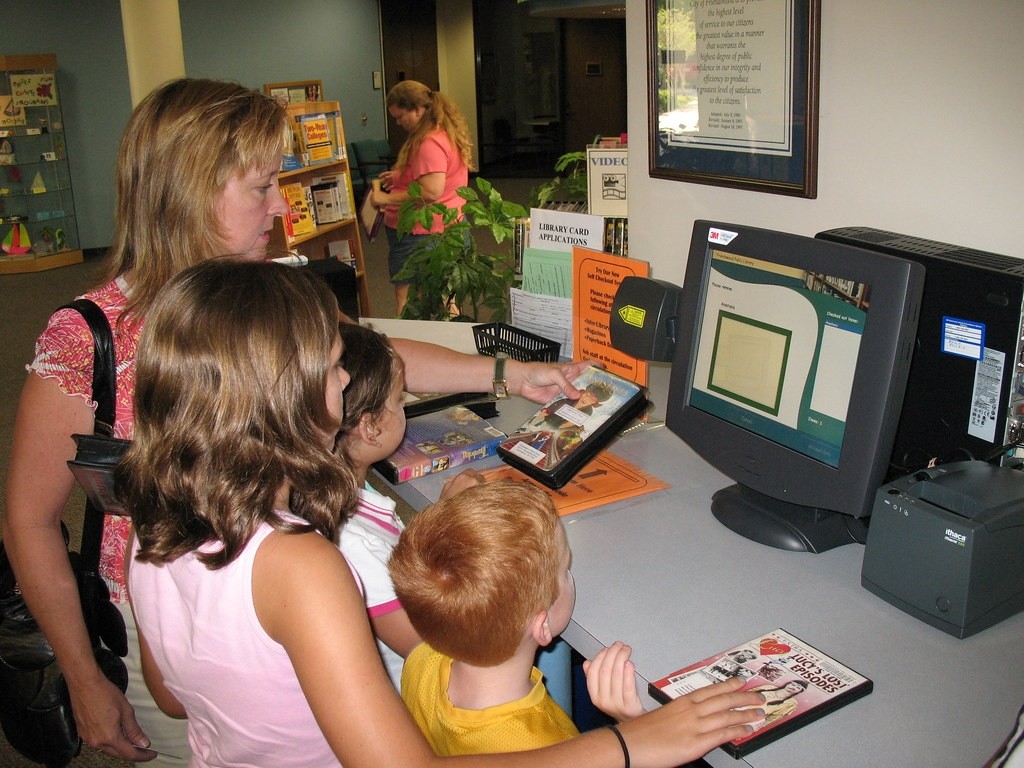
[646,0,822,200]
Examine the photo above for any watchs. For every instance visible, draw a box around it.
[492,358,511,400]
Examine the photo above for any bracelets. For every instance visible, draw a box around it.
[608,725,630,768]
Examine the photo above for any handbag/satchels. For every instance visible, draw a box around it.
[358,179,392,243]
[0,298,128,768]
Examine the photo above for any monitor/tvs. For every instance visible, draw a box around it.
[665,219,925,554]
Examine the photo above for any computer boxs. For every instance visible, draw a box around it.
[814,228,1024,470]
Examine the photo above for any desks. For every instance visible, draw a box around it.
[519,115,561,140]
[367,391,1024,768]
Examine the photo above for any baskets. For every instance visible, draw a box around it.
[472,321,562,364]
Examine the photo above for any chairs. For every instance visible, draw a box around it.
[491,117,513,152]
[346,138,398,211]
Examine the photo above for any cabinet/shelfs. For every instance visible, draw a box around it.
[0,52,84,274]
[267,101,373,317]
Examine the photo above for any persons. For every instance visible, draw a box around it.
[112,259,765,768]
[330,325,487,694]
[371,80,475,320]
[400,482,648,768]
[0,77,606,768]
[709,649,809,729]
[305,85,319,102]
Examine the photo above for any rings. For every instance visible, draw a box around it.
[97,749,102,753]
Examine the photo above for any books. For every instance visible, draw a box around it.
[288,89,305,103]
[278,112,357,272]
[305,85,321,102]
[270,88,289,103]
